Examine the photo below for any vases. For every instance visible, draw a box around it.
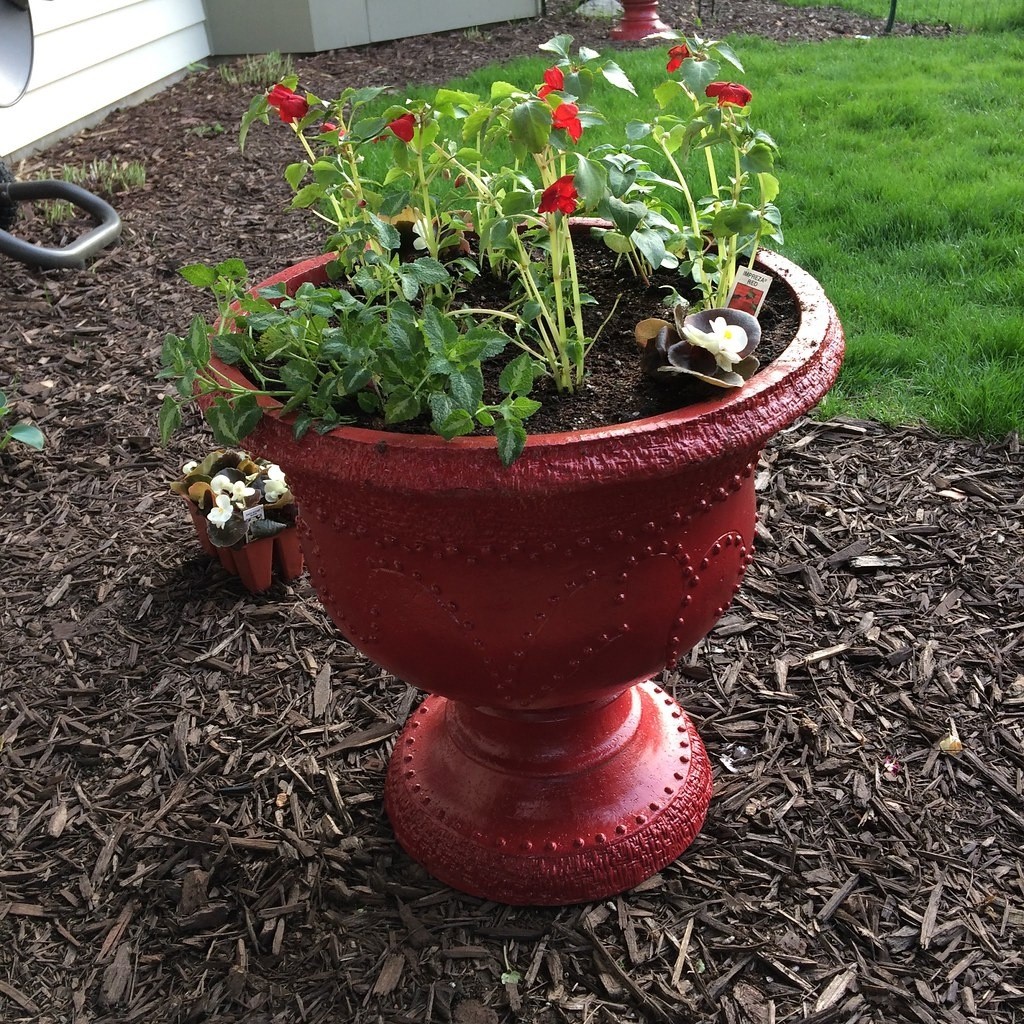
[197,205,853,904]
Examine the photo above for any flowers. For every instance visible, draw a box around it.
[169,445,297,545]
[147,12,800,460]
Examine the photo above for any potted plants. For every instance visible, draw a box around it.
[189,504,309,598]
